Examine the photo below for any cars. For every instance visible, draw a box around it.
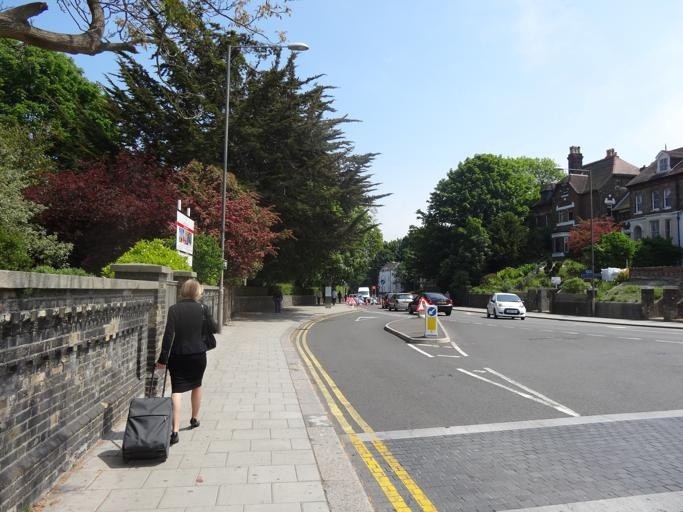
[485,292,528,322]
[346,287,457,316]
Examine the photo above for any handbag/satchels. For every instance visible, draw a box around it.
[201,318,217,351]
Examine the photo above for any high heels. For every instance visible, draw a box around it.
[171,430,178,446]
[190,418,200,429]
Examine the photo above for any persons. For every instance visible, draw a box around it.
[337,290,341,303]
[315,288,322,304]
[156,280,219,445]
[331,289,337,305]
[444,290,450,299]
[272,288,283,313]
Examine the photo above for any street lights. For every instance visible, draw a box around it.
[551,167,597,318]
[215,41,312,334]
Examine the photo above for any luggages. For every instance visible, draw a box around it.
[121,362,171,461]
